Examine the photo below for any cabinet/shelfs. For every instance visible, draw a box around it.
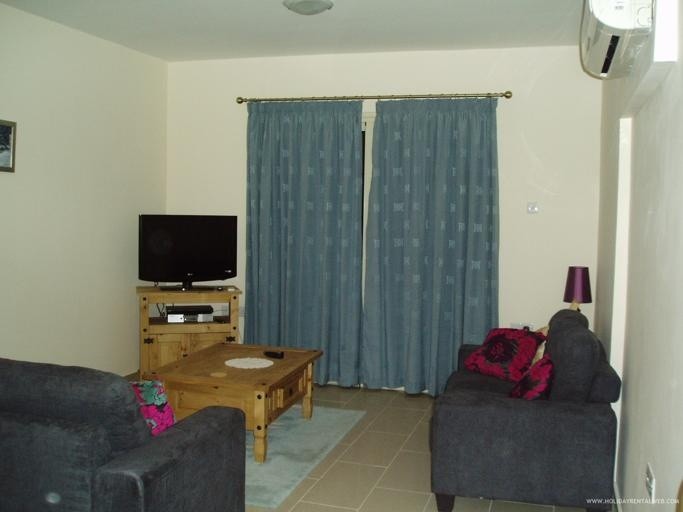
[135,286,242,381]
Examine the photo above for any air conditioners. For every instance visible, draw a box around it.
[577,0,655,83]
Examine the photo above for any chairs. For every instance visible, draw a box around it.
[0,357,246,511]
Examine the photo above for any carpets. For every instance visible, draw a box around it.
[244,404,367,510]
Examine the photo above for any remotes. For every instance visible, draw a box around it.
[266,352,283,358]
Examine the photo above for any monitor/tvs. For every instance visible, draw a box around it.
[139,214,237,291]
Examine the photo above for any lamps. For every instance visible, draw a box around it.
[562,266,592,311]
[283,0,333,17]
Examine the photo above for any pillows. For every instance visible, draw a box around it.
[127,380,173,435]
[463,326,554,401]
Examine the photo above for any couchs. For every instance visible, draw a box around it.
[427,308,622,512]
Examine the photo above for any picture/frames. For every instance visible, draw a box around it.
[0,119,16,173]
